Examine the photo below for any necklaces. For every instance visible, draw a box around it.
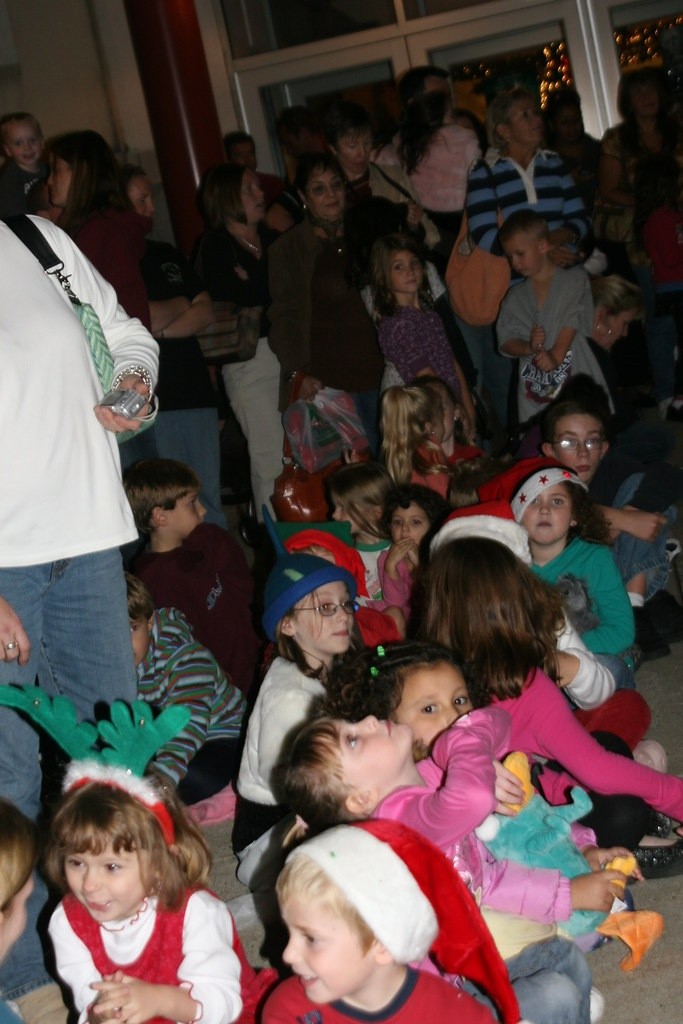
[240,236,261,253]
[318,227,347,253]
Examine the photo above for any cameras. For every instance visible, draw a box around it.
[100,388,148,421]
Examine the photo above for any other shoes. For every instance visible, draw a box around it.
[634,836,683,878]
[633,590,682,660]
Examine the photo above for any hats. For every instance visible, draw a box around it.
[428,497,532,567]
[475,455,588,523]
[286,819,523,1024]
[262,552,358,643]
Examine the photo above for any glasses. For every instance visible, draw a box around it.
[287,600,355,617]
[298,180,343,197]
[550,438,603,450]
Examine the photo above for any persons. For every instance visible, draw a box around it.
[0,66,683,1024]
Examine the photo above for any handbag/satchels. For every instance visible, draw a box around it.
[272,368,369,523]
[443,160,512,326]
[196,302,260,365]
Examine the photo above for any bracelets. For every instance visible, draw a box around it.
[160,327,164,339]
[114,366,151,402]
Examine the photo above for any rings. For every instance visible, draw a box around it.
[4,642,18,650]
